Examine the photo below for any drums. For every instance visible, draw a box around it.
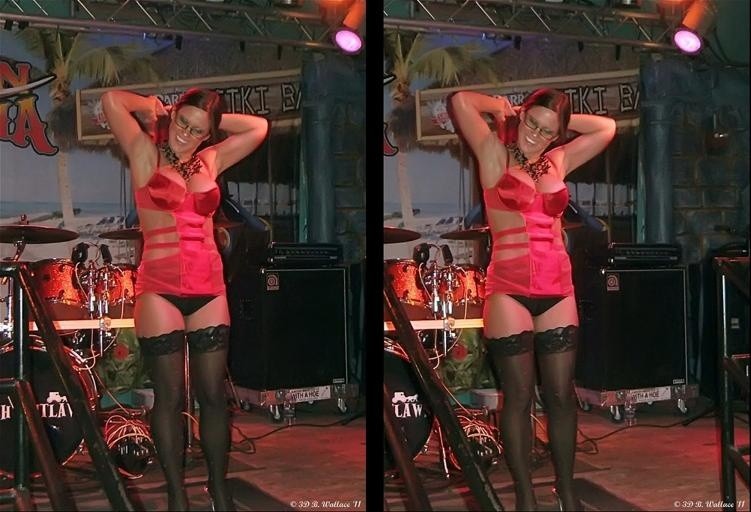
[436,263,486,322]
[24,257,92,323]
[96,259,138,321]
[381,339,435,475]
[382,256,441,322]
[0,335,99,479]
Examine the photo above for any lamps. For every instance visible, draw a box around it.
[335,0,367,56]
[670,1,718,60]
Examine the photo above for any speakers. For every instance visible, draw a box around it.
[698,256,751,413]
[223,265,350,407]
[572,265,691,407]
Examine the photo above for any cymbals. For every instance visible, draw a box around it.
[98,227,143,240]
[562,222,589,229]
[0,223,80,245]
[212,221,242,231]
[382,226,423,244]
[437,225,490,239]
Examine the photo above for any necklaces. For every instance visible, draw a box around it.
[504,143,551,184]
[159,144,202,182]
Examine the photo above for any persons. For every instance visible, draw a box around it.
[450,87,616,512]
[101,87,268,512]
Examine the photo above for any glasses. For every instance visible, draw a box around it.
[523,111,557,141]
[174,112,206,140]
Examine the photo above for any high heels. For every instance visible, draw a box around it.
[552,487,563,511]
[204,483,216,511]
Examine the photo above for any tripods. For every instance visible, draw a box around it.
[415,261,461,480]
